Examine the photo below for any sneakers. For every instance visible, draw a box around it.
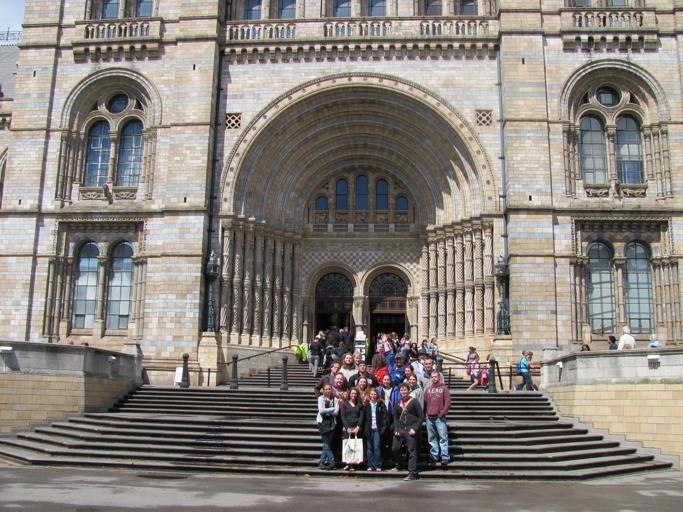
[319,460,448,480]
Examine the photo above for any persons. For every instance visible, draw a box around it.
[526,352,542,393]
[580,344,590,351]
[646,334,664,348]
[305,324,451,481]
[616,325,637,350]
[482,353,495,392]
[607,336,617,349]
[480,366,487,391]
[463,346,479,385]
[464,354,479,390]
[512,349,541,391]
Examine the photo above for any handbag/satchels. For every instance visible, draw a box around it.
[318,418,336,435]
[342,434,363,464]
